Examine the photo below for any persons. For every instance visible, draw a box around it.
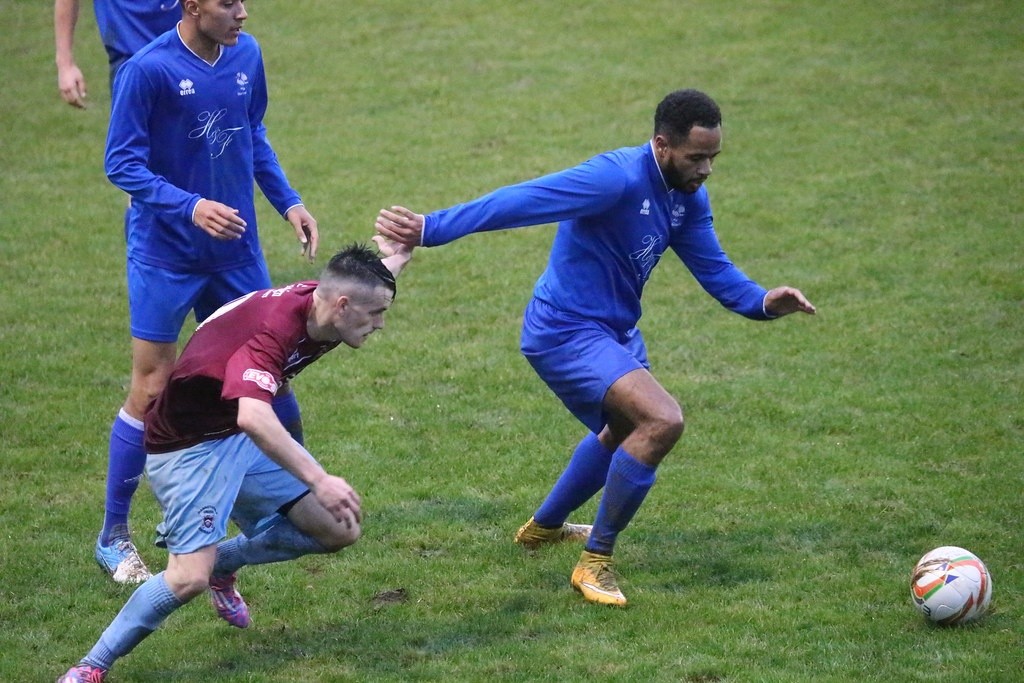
[55,1,185,109]
[95,0,318,584]
[53,234,414,683]
[376,89,818,609]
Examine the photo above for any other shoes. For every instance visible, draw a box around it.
[155,535,167,547]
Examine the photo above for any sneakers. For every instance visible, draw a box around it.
[515,516,593,543]
[571,552,627,606]
[210,573,248,628]
[95,531,154,583]
[57,667,107,683]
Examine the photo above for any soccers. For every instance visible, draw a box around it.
[910,546,993,624]
